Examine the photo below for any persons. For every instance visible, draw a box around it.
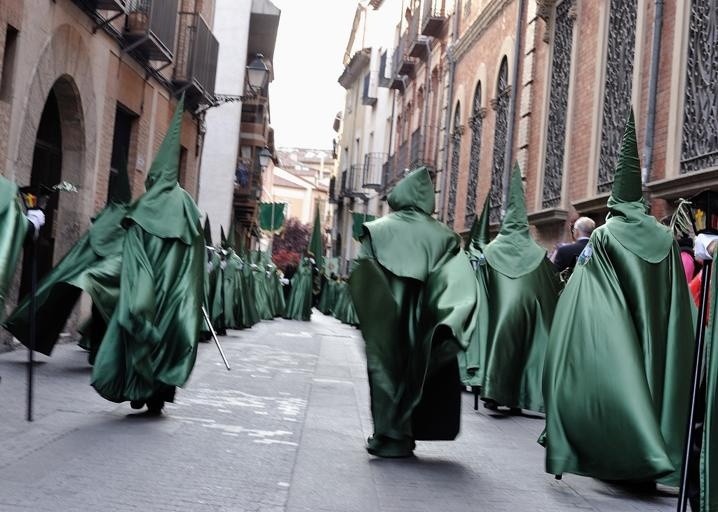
[284,246,314,321]
[93,92,206,417]
[472,158,553,413]
[194,212,286,335]
[1,173,25,306]
[311,254,367,331]
[5,141,130,363]
[553,216,595,296]
[346,167,479,458]
[456,180,490,392]
[537,104,696,498]
[687,232,718,512]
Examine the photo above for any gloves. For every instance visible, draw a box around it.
[24,202,48,229]
[690,230,717,265]
